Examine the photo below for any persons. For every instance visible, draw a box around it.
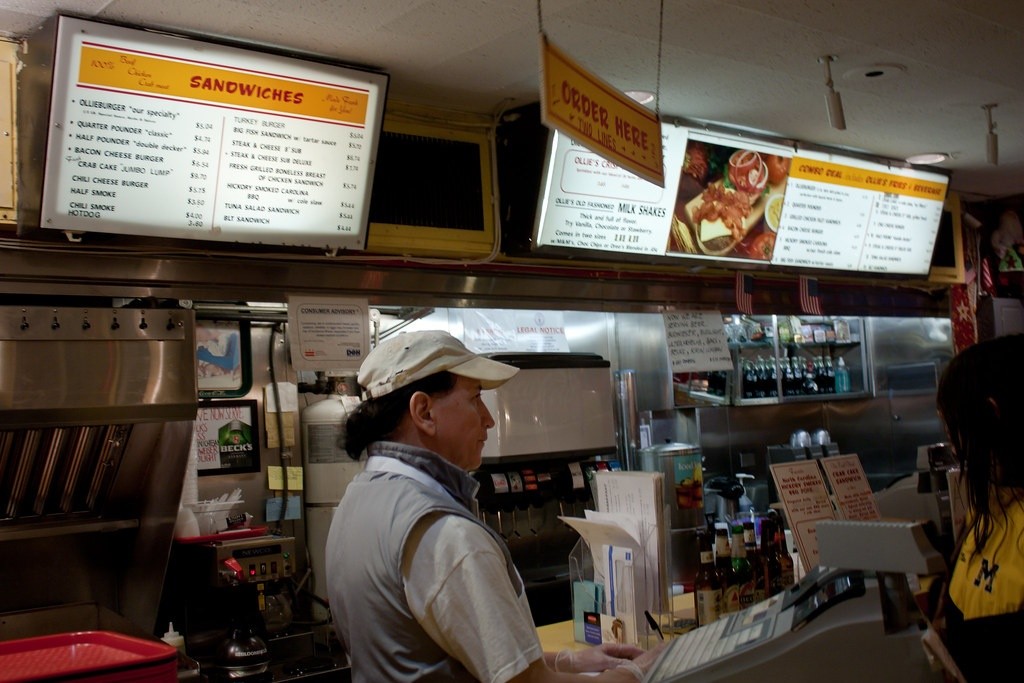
[326,330,664,683]
[936,332,1024,683]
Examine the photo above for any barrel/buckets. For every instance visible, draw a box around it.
[637,438,705,530]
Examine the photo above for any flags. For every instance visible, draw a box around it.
[964,245,996,296]
[800,276,820,314]
[736,271,753,315]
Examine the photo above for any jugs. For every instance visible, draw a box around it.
[703,475,744,524]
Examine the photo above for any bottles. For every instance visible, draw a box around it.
[161,621,186,655]
[694,516,794,628]
[741,356,851,398]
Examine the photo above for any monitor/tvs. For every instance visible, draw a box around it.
[366,122,495,258]
[902,190,968,283]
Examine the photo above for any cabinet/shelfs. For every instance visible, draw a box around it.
[671,311,871,405]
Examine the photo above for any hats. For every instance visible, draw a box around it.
[356,328,520,403]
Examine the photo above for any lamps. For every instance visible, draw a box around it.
[982,101,999,164]
[818,56,847,131]
